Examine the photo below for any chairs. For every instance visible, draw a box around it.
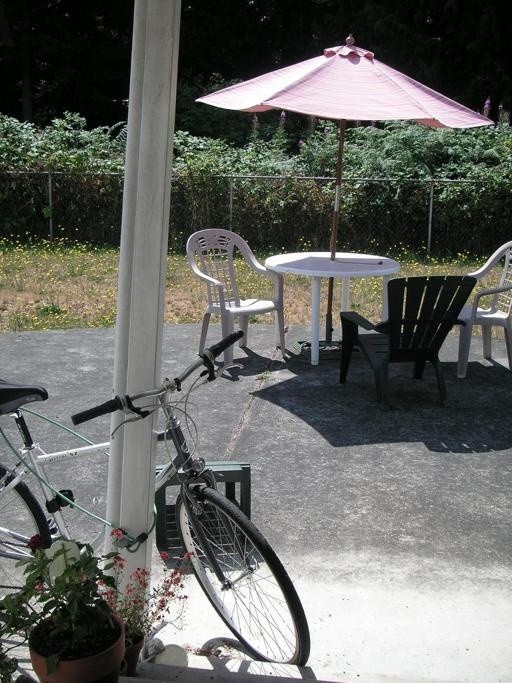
[338,275,477,411]
[442,239,512,378]
[185,228,287,366]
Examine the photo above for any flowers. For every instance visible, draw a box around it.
[35,529,196,640]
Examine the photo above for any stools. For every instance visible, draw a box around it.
[155,461,252,550]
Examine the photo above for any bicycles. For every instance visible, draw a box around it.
[0,328,310,667]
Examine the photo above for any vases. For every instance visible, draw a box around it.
[116,630,147,677]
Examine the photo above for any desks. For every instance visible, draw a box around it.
[265,251,401,364]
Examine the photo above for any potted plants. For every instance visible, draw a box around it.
[0,540,126,683]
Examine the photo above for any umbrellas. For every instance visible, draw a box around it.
[196,32,497,343]
